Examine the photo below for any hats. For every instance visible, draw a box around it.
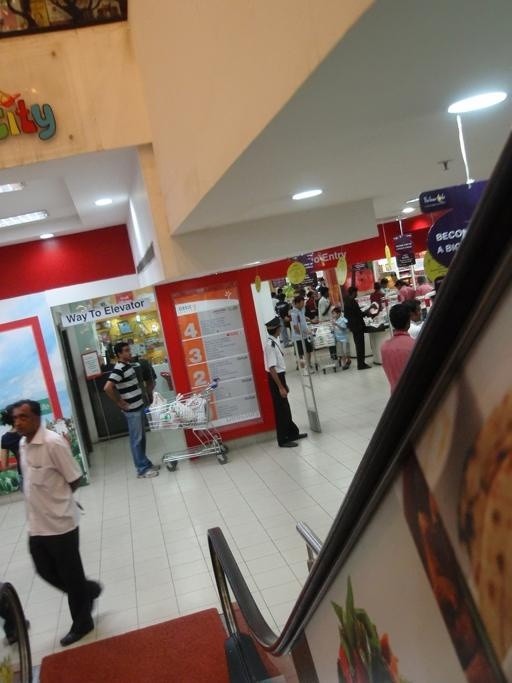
[265,316,284,329]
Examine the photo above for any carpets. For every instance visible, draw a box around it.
[37,606,229,682]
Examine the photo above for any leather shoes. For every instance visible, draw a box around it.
[89,583,101,615]
[283,440,298,446]
[60,622,93,646]
[294,432,307,441]
[8,619,28,643]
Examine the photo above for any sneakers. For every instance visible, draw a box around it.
[302,367,309,376]
[136,468,159,478]
[148,464,160,471]
[345,360,351,369]
[308,365,315,374]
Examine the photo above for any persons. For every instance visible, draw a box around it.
[271,271,431,400]
[10,400,102,646]
[263,316,308,448]
[0,407,24,493]
[105,342,162,479]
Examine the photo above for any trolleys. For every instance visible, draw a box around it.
[304,318,339,373]
[144,376,230,470]
